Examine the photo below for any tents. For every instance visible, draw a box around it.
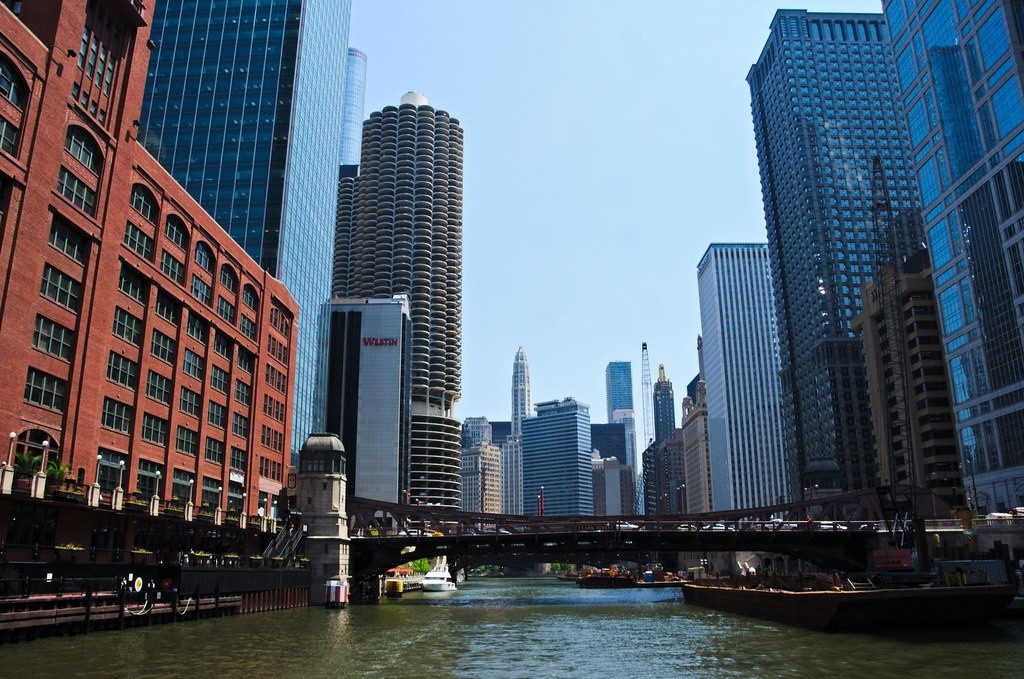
[986,513,1013,525]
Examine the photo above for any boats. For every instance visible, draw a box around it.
[418,555,458,591]
[555,562,695,587]
[682,574,1018,632]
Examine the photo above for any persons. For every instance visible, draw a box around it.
[807,516,813,528]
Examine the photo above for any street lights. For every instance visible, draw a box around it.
[263,498,268,516]
[155,471,161,495]
[40,440,50,471]
[273,500,277,518]
[94,454,102,483]
[118,460,124,487]
[189,479,194,503]
[676,483,685,513]
[217,486,222,507]
[242,493,247,513]
[659,493,668,514]
[540,485,545,516]
[7,431,17,465]
[537,495,540,517]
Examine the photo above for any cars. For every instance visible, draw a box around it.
[398,524,521,536]
[606,520,639,530]
[678,518,882,531]
